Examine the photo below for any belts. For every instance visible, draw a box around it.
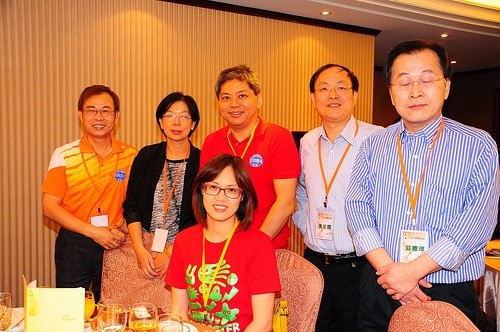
[306,246,365,266]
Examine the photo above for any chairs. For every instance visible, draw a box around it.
[99,231,174,311]
[275,249,325,332]
[388,301,481,332]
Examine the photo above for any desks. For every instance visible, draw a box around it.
[470,237,500,332]
[0,298,213,332]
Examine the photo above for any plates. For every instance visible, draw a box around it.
[159,321,198,332]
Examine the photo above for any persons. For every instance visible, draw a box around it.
[164,154,283,332]
[122,92,202,279]
[190,64,300,250]
[42,85,139,303]
[344,40,500,332]
[292,64,386,332]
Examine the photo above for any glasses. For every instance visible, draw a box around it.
[162,111,193,123]
[314,86,354,95]
[389,76,445,91]
[202,182,243,200]
[81,106,117,116]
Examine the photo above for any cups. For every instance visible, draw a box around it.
[84,291,95,322]
[129,302,189,332]
[97,299,127,332]
[0,293,12,331]
